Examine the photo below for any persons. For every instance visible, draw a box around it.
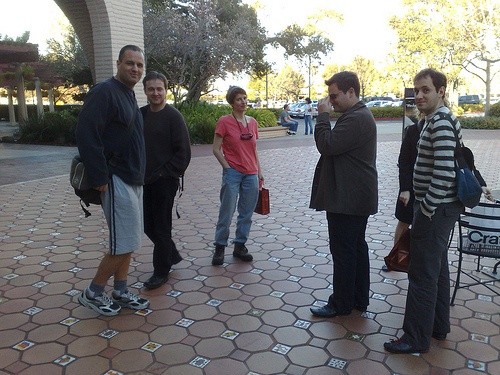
[382,97,490,271]
[281,104,299,136]
[138,71,191,288]
[212,85,264,265]
[304,98,313,135]
[383,69,462,352]
[310,71,379,317]
[76,45,151,316]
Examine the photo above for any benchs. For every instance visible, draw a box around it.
[255,121,289,140]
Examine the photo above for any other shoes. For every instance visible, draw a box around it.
[212,246,225,265]
[143,269,169,289]
[287,131,296,136]
[383,338,429,355]
[310,303,352,318]
[167,252,184,271]
[352,305,367,313]
[382,263,392,272]
[432,331,447,341]
[233,243,253,262]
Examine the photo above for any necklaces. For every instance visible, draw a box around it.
[232,111,253,141]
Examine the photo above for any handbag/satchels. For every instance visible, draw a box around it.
[254,187,270,216]
[455,144,482,208]
[384,227,412,273]
[70,156,103,205]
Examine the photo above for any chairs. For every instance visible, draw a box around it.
[449,202,500,306]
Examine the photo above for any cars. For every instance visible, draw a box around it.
[457,94,500,109]
[245,94,404,120]
[205,98,230,106]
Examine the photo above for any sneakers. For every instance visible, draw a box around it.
[78,285,122,316]
[110,290,150,311]
